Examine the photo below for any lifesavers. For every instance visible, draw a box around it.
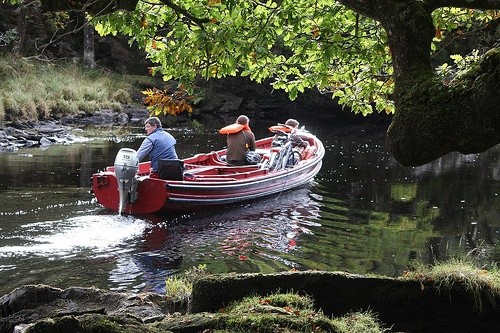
[268,126,296,133]
[219,124,251,147]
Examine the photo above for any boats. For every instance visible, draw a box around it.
[90,127,325,216]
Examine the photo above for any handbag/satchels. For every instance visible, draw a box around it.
[158,159,185,181]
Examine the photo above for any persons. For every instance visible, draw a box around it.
[137,117,178,173]
[225,115,258,166]
[268,119,309,147]
